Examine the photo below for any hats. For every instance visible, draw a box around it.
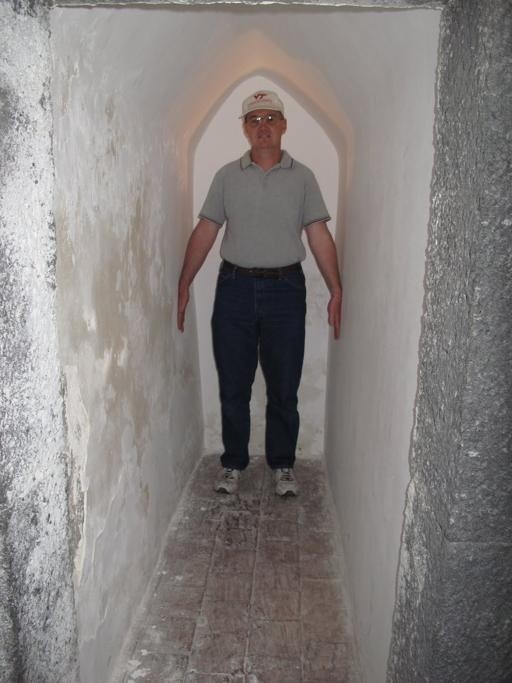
[237,89,285,119]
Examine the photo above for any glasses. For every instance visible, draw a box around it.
[245,113,282,128]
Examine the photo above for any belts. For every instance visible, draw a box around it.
[222,261,302,278]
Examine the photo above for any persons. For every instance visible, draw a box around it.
[178,90,342,497]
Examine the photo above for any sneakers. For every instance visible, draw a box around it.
[214,465,242,495]
[272,466,301,496]
